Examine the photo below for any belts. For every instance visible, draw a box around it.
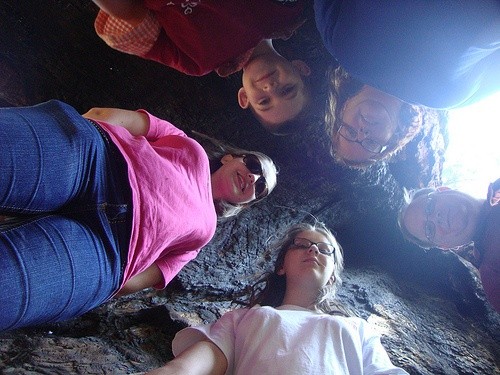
[88,118,133,276]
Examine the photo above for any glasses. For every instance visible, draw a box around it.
[242,154,268,199]
[336,117,389,154]
[290,236,336,259]
[424,190,437,247]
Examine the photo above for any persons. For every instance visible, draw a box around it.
[94,0,314,136]
[0,100,277,330]
[398,177,500,315]
[141,223,409,375]
[314,0,500,169]
